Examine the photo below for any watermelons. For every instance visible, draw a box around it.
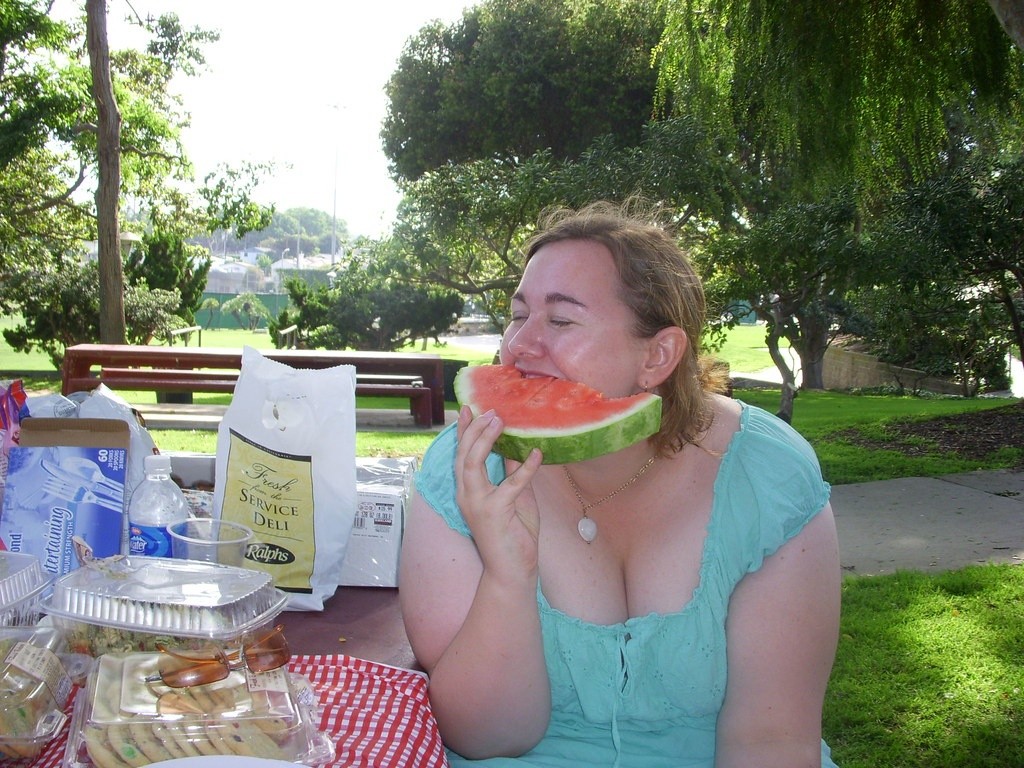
[453,365,662,465]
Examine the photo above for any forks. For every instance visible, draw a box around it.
[42,477,123,515]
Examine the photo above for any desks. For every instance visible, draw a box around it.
[61,343,444,425]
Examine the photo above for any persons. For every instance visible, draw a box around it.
[400,188,842,767]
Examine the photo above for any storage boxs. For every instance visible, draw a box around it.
[0,418,130,600]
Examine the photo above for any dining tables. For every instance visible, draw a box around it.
[274,585,427,673]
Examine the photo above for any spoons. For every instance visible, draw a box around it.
[61,456,124,491]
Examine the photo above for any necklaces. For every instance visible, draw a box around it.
[562,449,662,543]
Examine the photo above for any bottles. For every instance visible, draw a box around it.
[127,454,189,562]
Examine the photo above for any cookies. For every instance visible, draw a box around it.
[86,652,288,768]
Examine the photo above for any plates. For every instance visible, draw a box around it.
[138,755,314,768]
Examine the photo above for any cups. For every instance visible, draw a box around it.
[167,518,251,568]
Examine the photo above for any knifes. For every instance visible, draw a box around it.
[42,460,123,502]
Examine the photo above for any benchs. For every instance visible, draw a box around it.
[67,368,433,428]
